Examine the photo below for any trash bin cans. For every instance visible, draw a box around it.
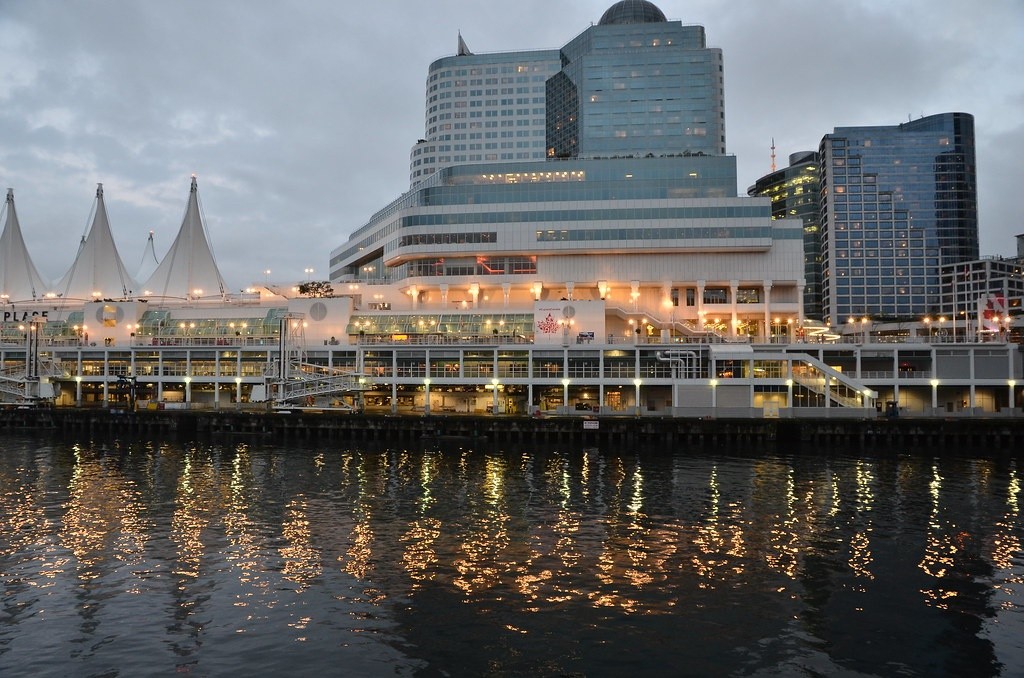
[885,401,899,418]
